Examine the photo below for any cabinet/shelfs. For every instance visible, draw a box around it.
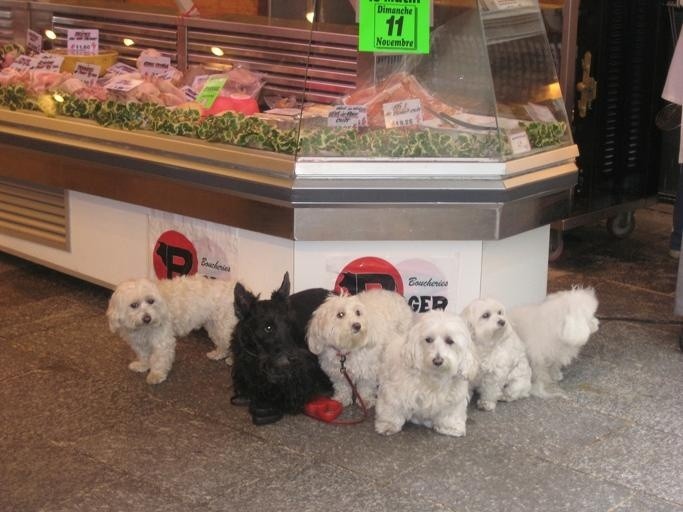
[0,0,578,328]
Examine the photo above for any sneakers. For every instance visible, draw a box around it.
[668,232,683,258]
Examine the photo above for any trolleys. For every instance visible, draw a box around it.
[549,0,679,267]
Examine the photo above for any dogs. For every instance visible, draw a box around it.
[459,297,533,412]
[304,287,414,411]
[227,270,344,427]
[374,307,476,437]
[505,283,600,401]
[105,272,240,385]
